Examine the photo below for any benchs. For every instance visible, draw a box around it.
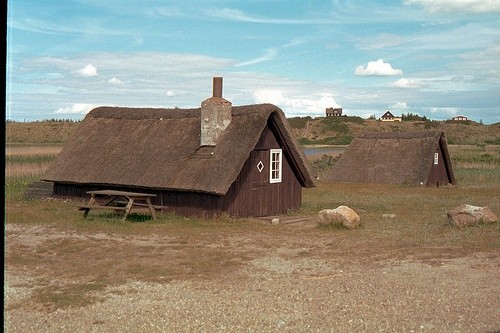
[78,205,129,211]
[113,201,168,218]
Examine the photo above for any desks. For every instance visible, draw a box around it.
[83,190,158,222]
